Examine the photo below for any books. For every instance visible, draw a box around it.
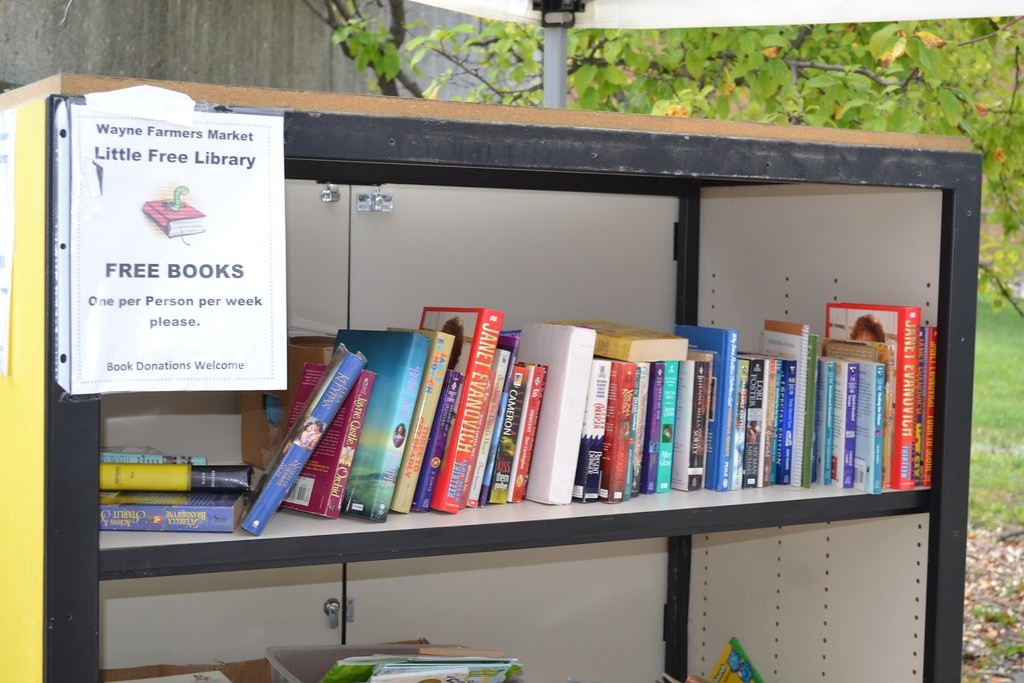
[319,646,524,683]
[98,303,936,536]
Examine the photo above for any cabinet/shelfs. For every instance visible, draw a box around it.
[0,75,981,683]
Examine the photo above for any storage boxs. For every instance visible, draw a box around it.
[98,638,523,683]
[240,334,337,470]
